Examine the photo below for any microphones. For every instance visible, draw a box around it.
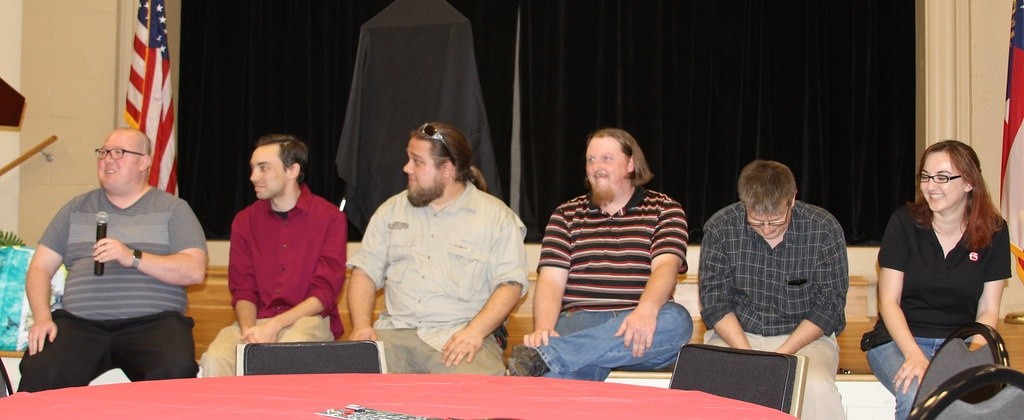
[94,211,110,276]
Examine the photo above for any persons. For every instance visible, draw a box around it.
[861,140,1011,420]
[509,129,693,382]
[698,161,849,420]
[200,136,346,378]
[18,128,207,393]
[347,123,529,374]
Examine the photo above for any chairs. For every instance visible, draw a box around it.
[903,322,1024,420]
[235,341,388,375]
[668,343,809,420]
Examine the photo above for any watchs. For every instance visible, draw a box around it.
[130,248,142,269]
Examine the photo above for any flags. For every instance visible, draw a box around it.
[126,1,179,198]
[1000,0,1024,283]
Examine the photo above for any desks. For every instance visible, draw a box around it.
[0,372,794,420]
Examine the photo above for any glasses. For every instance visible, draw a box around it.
[916,174,961,183]
[744,200,789,226]
[95,149,144,159]
[419,122,458,165]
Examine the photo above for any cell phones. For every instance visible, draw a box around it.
[788,279,807,286]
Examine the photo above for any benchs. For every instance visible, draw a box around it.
[181,265,1024,378]
